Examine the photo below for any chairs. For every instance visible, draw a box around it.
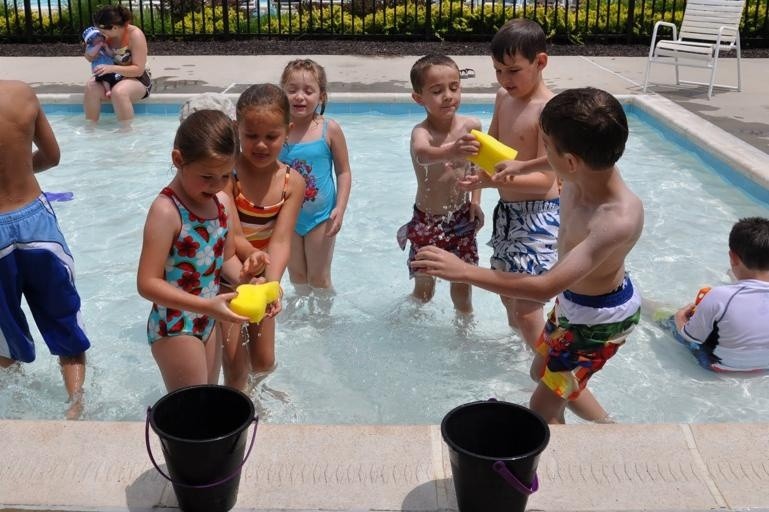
[644,0,748,99]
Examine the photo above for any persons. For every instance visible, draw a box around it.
[82,5,153,124]
[276,58,351,292]
[397,52,482,313]
[651,217,769,375]
[455,18,559,354]
[83,27,127,97]
[136,109,262,396]
[214,83,307,391]
[409,86,645,427]
[0,78,91,393]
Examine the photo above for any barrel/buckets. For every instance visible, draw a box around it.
[439,398,550,512]
[144,383,259,512]
